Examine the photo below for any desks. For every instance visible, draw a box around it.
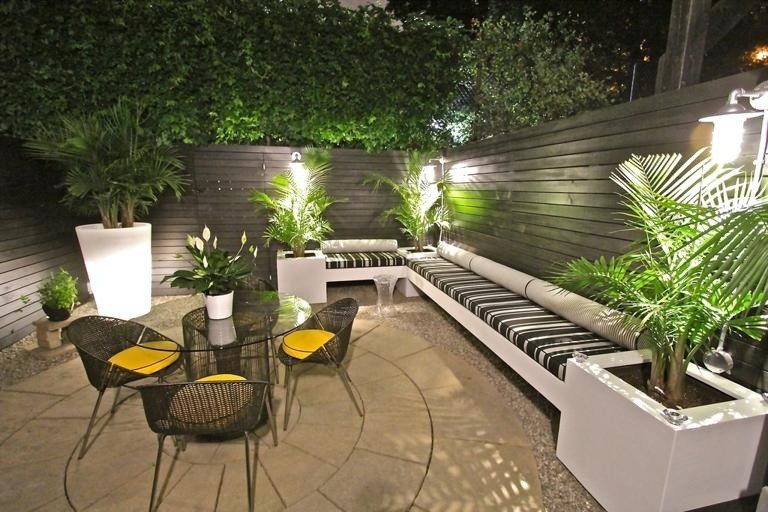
[125,292,311,406]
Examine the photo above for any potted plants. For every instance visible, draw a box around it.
[24,87,191,322]
[248,140,352,306]
[539,143,768,512]
[39,268,78,321]
[355,146,466,298]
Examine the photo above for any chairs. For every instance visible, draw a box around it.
[68,315,184,459]
[273,298,365,431]
[137,374,269,512]
[206,280,280,386]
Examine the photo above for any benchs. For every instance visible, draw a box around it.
[319,238,408,297]
[407,240,655,413]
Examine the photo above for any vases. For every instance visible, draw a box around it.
[206,290,234,321]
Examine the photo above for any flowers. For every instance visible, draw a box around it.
[159,220,264,289]
